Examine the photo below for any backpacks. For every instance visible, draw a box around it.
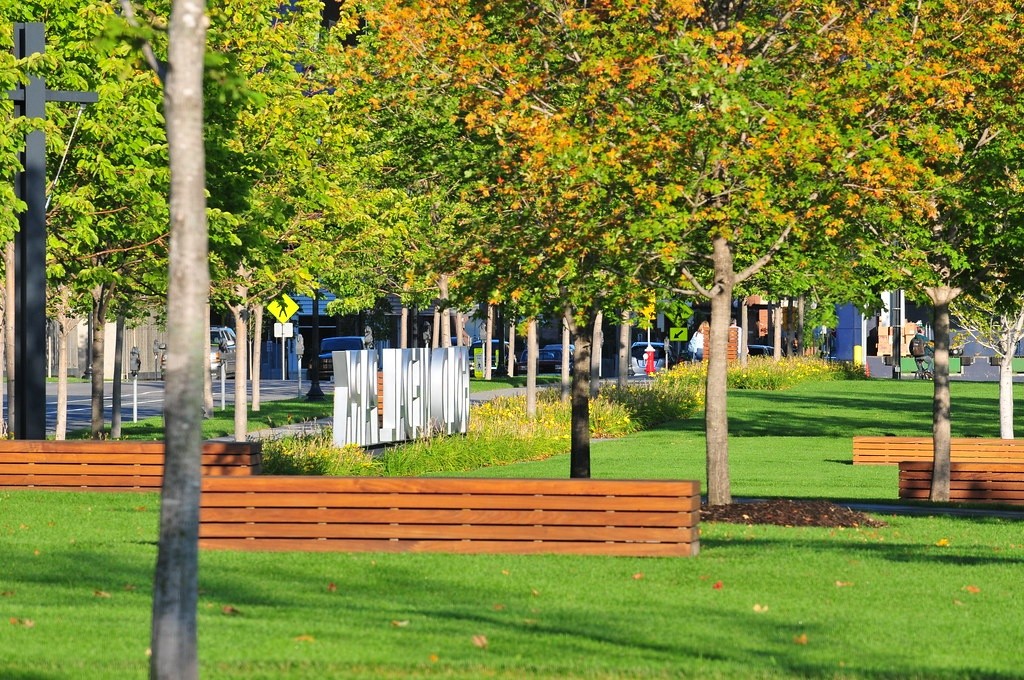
[909,338,925,357]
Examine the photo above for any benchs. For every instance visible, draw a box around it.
[853,435,1024,466]
[198,475,701,559]
[0,441,263,492]
[898,461,1024,505]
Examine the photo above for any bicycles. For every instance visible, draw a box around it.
[911,357,935,380]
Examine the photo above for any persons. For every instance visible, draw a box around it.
[913,329,934,379]
[729,318,749,359]
[693,321,709,361]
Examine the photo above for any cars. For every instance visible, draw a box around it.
[747,345,785,358]
[517,343,576,375]
[468,340,518,376]
[307,336,365,380]
[628,342,681,376]
[159,326,236,380]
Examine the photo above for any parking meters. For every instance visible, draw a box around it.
[129,346,140,423]
[421,321,432,349]
[598,331,604,378]
[295,336,305,399]
[365,327,373,351]
[664,338,671,373]
[153,339,159,382]
[217,337,228,410]
[480,322,487,378]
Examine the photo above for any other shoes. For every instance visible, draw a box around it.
[925,371,934,379]
